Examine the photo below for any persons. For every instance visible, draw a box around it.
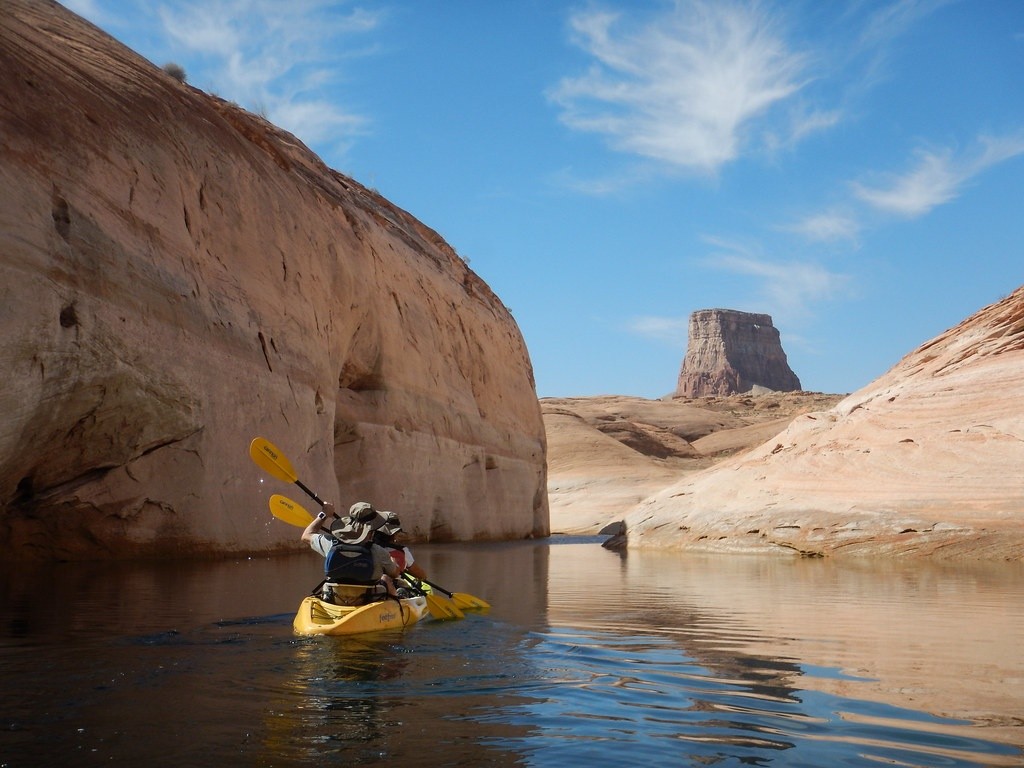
[301,501,400,602]
[372,510,426,587]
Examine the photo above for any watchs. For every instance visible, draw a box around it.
[318,511,327,520]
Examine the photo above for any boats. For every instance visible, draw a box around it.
[291,571,435,638]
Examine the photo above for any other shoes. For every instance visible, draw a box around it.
[396,588,408,599]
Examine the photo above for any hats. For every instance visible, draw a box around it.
[377,512,402,535]
[330,501,389,545]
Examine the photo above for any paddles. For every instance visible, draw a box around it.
[268,494,491,610]
[249,436,465,621]
